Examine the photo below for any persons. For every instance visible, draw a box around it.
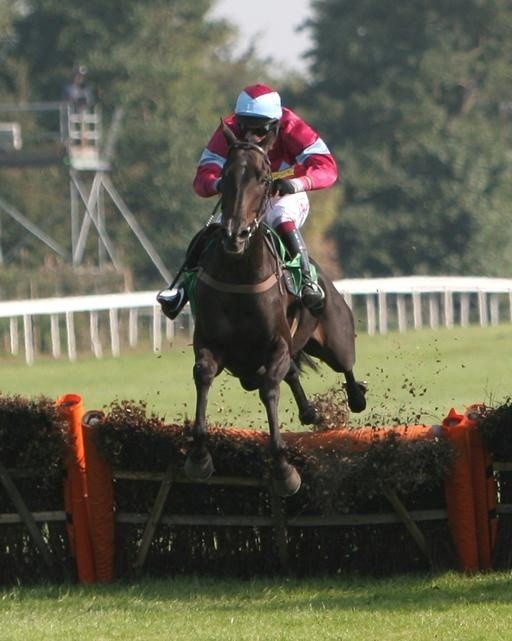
[154,82,339,317]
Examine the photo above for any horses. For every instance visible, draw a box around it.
[176,114,367,498]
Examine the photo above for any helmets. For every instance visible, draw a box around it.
[235,83,283,120]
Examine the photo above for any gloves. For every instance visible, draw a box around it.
[270,178,295,197]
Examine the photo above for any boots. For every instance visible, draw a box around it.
[282,228,325,311]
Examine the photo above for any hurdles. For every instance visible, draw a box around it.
[2,393,511,588]
